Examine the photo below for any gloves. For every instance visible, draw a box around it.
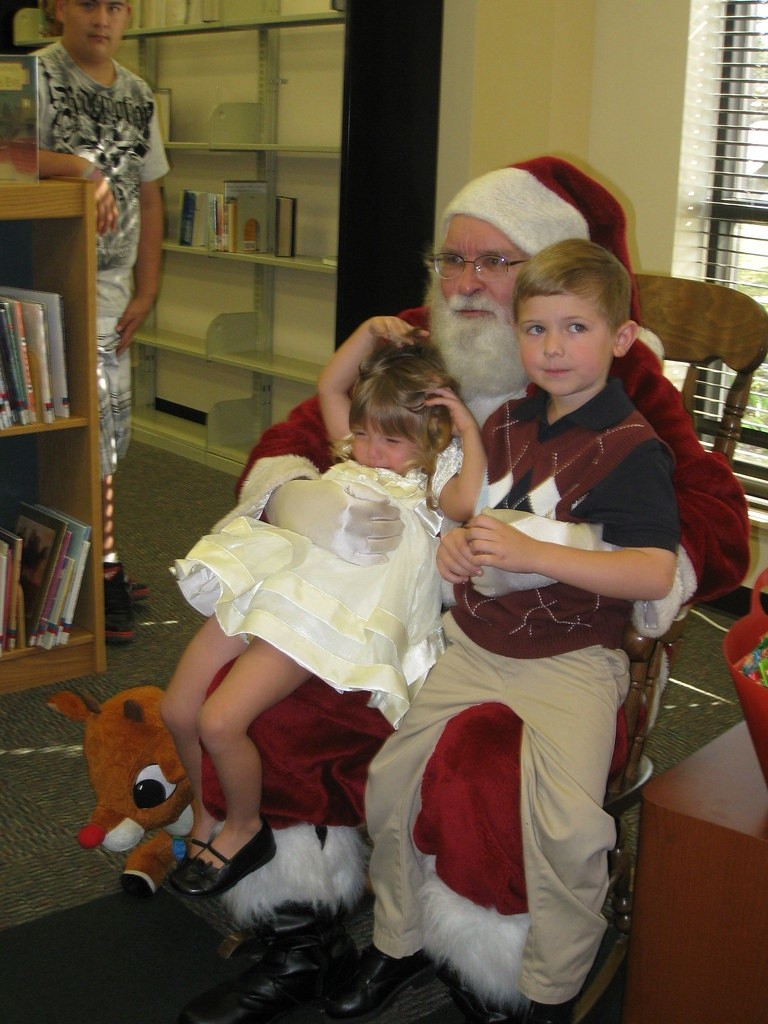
[267,479,404,565]
[471,508,605,596]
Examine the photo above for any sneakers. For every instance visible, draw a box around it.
[124,578,148,600]
[106,586,135,642]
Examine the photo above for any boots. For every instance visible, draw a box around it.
[176,825,365,1024]
[425,873,531,1024]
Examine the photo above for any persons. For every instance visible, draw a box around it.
[204,155,749,1024]
[324,238,685,1024]
[162,314,486,899]
[31,1,171,652]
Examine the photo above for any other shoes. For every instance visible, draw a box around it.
[168,819,277,900]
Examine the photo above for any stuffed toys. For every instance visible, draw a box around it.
[54,684,196,899]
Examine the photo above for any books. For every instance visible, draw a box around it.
[1,498,93,654]
[208,178,297,258]
[0,285,71,430]
[1,54,40,185]
[178,189,205,246]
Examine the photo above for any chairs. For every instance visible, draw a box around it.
[94,270,768,1024]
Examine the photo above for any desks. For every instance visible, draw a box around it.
[617,718,768,1024]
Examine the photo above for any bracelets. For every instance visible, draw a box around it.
[78,162,101,182]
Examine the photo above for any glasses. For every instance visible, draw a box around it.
[430,252,528,282]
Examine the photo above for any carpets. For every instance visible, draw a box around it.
[0,885,326,1024]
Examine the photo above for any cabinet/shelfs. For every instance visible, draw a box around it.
[0,175,108,698]
[13,0,445,481]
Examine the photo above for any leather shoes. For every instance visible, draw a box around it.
[322,947,435,1021]
[527,996,579,1024]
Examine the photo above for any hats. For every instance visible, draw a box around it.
[440,154,666,363]
